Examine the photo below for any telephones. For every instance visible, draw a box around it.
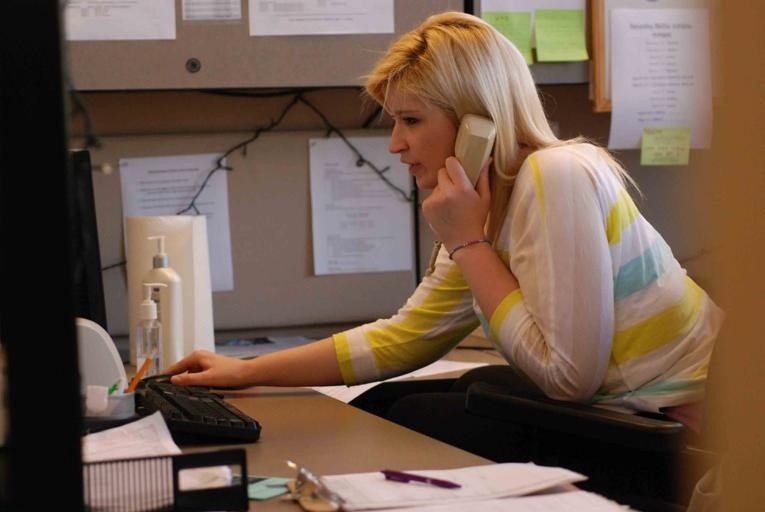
[428,113,498,235]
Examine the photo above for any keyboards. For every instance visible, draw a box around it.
[130,377,263,444]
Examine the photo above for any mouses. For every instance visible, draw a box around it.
[143,372,180,384]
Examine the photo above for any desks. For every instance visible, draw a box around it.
[0,386,640,512]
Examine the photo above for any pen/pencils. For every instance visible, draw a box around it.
[106,356,152,395]
[381,469,463,489]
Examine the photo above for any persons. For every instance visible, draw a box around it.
[163,10,724,511]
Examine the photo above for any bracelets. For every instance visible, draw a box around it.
[448,238,493,260]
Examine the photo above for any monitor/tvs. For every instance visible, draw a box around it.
[69,148,110,330]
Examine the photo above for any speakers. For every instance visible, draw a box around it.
[75,315,128,416]
